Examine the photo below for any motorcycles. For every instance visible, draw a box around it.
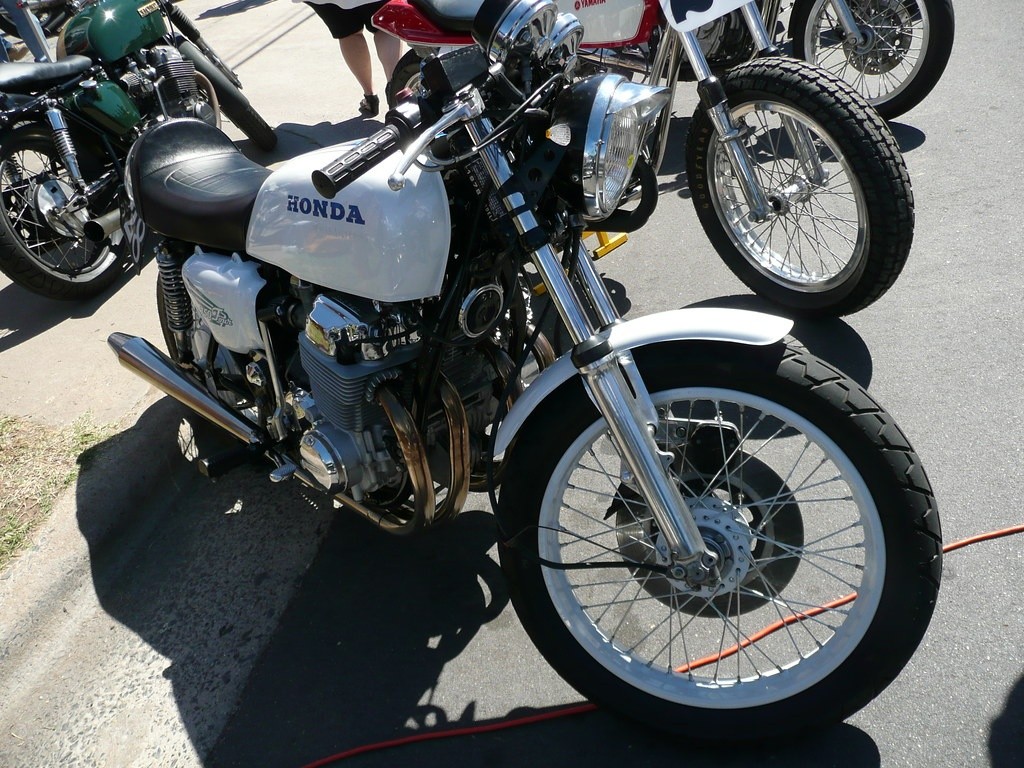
[0,1,277,303]
[108,0,955,746]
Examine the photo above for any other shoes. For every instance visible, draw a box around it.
[360,94,380,114]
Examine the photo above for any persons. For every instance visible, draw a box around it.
[292,0,400,116]
[0,33,29,64]
